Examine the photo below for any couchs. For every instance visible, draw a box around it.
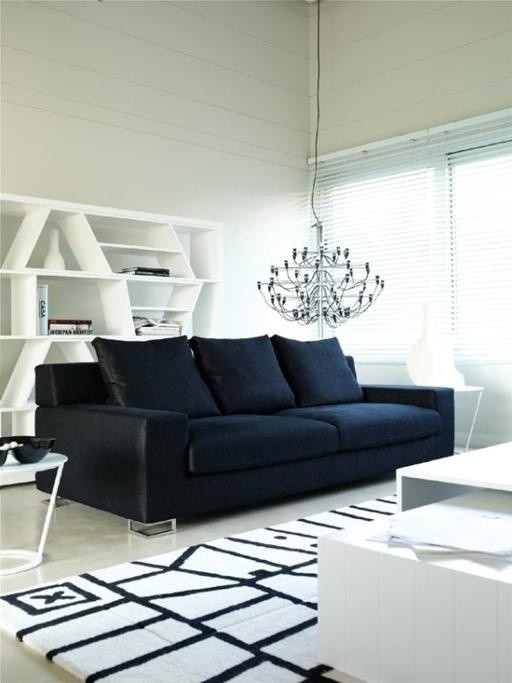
[31,356,454,538]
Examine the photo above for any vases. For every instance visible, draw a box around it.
[408,302,453,387]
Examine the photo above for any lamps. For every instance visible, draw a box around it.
[258,221,384,338]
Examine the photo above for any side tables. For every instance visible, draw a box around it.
[0,452,69,576]
[451,386,486,451]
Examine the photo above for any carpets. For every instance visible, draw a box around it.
[0,490,404,683]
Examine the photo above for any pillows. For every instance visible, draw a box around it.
[270,335,364,408]
[188,333,300,415]
[91,336,222,418]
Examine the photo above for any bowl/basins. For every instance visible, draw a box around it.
[11,437,57,464]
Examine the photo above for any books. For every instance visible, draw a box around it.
[132,314,183,336]
[119,270,169,276]
[122,266,169,272]
[48,319,95,335]
[367,510,511,559]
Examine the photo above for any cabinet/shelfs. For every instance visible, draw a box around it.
[0,194,223,487]
